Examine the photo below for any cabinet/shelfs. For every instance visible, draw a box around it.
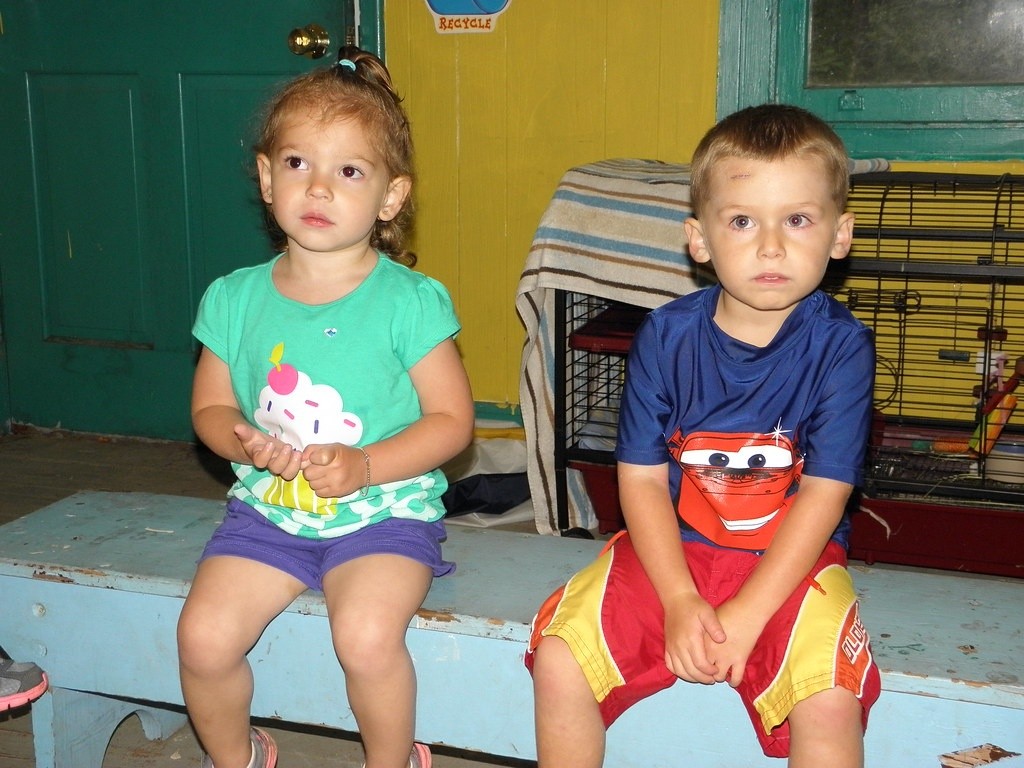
[553,289,651,534]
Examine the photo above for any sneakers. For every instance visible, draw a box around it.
[0,656,47,714]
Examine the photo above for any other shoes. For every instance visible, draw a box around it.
[201,728,279,768]
[361,742,433,768]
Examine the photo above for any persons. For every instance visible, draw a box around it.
[176,46,475,768]
[524,104,877,768]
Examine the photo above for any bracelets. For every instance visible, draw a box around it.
[356,446,370,497]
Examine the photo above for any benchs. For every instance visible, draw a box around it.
[0,488,1023,768]
